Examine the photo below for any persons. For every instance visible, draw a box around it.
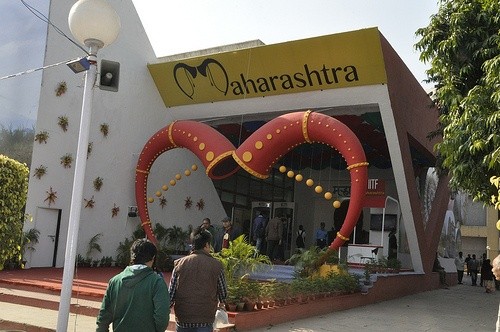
[95,238,171,332]
[432,252,500,332]
[167,228,228,332]
[187,211,328,264]
[388,228,397,259]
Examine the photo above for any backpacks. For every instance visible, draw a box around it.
[295,230,304,248]
[256,218,265,238]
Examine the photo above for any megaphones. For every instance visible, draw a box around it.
[98,59,120,92]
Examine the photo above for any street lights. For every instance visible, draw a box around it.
[56,1,121,332]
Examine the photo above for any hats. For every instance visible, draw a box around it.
[222,217,231,222]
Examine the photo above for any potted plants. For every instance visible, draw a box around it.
[0,224,41,271]
[206,235,362,310]
[74,232,114,269]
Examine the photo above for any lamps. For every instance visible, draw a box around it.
[66,56,90,74]
[369,256,402,274]
